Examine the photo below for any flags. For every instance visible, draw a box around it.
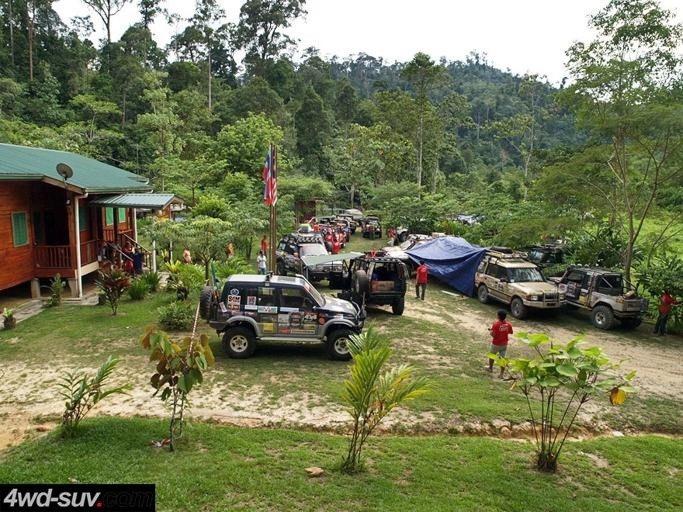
[262,144,278,208]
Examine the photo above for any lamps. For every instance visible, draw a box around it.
[55,162,73,207]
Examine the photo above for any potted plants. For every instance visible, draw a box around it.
[1,307,17,328]
[39,272,66,306]
[95,286,106,305]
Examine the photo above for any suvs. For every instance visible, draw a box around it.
[275,209,407,316]
[199,270,366,361]
[547,266,649,331]
[475,249,566,320]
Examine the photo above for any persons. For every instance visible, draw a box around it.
[257,250,266,275]
[387,227,396,247]
[368,247,378,257]
[366,223,370,238]
[162,246,169,263]
[486,310,514,378]
[262,235,268,258]
[314,223,347,253]
[415,259,429,300]
[653,289,678,336]
[183,245,191,264]
[102,239,144,274]
[225,239,234,258]
